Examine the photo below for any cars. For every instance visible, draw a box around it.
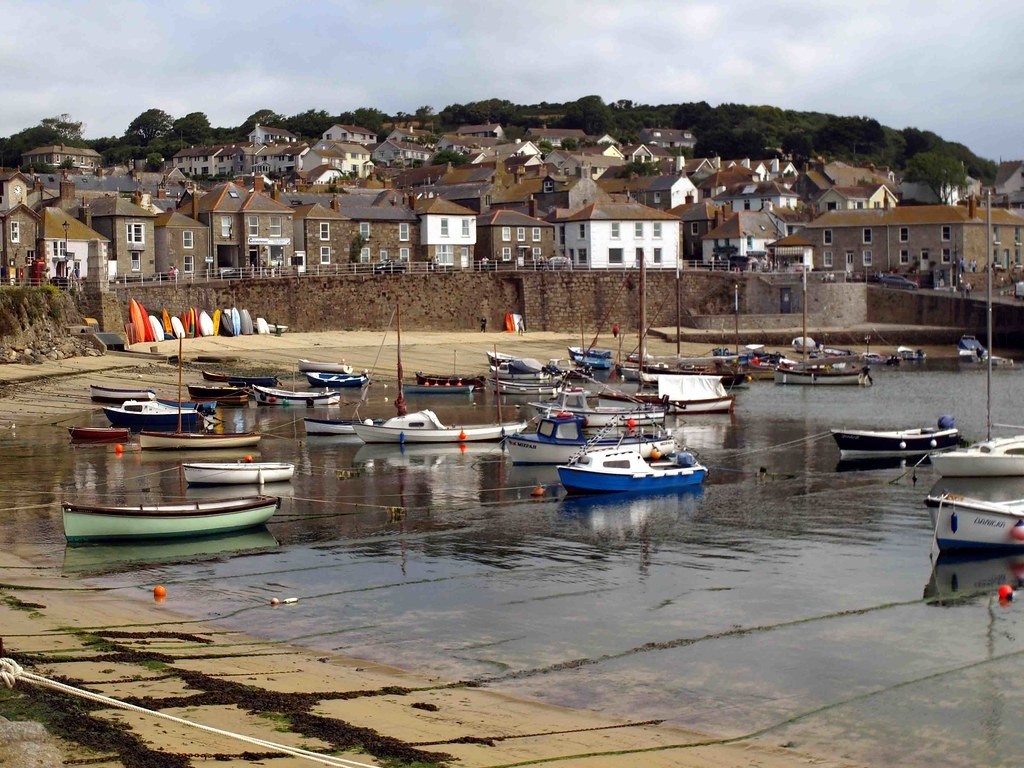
[223,268,252,279]
[881,275,919,290]
[1014,281,1024,301]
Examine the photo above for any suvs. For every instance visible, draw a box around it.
[729,256,770,272]
[374,258,407,274]
[534,256,570,270]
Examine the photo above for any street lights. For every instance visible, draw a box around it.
[62,220,70,288]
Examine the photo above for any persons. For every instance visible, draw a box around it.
[961,257,988,272]
[480,316,487,333]
[45,266,80,285]
[482,255,489,272]
[168,266,179,282]
[709,256,789,271]
[537,255,550,271]
[961,281,972,299]
[517,318,524,336]
[914,275,921,286]
[615,363,624,386]
[822,268,886,283]
[428,254,438,272]
[260,258,277,278]
[565,257,571,270]
[612,323,619,339]
[976,346,984,363]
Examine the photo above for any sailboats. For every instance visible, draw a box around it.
[139,330,263,452]
[400,245,1014,427]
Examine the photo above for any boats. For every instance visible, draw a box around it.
[301,416,386,438]
[555,404,711,495]
[183,358,370,409]
[61,494,283,544]
[830,414,962,462]
[182,460,295,488]
[89,384,156,404]
[100,400,227,434]
[123,297,290,346]
[350,303,528,445]
[66,424,132,444]
[923,492,1024,566]
[499,386,676,468]
[928,433,1024,478]
